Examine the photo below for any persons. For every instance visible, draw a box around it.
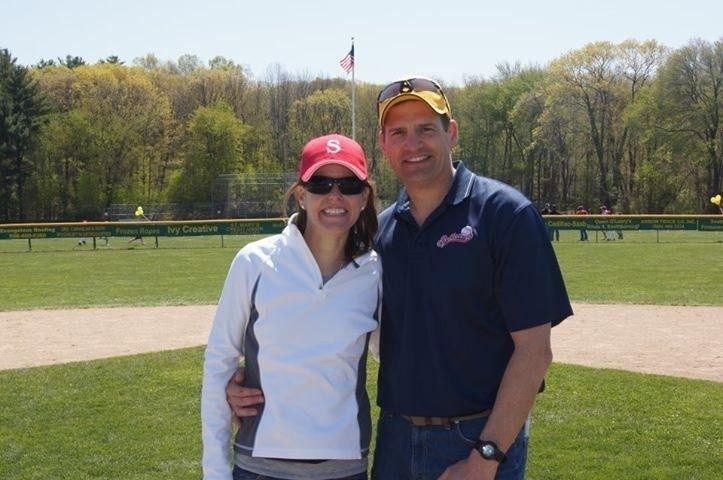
[127,236,145,245]
[224,76,574,479]
[616,212,623,240]
[95,220,108,246]
[605,209,615,240]
[575,206,588,240]
[599,206,606,240]
[548,204,561,241]
[199,134,382,480]
[71,238,93,251]
[540,202,550,214]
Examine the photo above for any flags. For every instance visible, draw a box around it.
[338,46,354,75]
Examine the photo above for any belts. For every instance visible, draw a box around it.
[398,408,493,428]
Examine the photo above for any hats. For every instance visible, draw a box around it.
[299,133,368,183]
[376,76,452,130]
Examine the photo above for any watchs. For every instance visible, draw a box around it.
[471,438,508,465]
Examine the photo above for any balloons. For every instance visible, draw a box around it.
[715,194,720,200]
[137,206,141,211]
[138,210,142,214]
[715,200,719,205]
[134,211,139,215]
[710,197,715,203]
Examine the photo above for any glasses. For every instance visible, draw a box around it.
[299,176,368,195]
[375,77,450,119]
